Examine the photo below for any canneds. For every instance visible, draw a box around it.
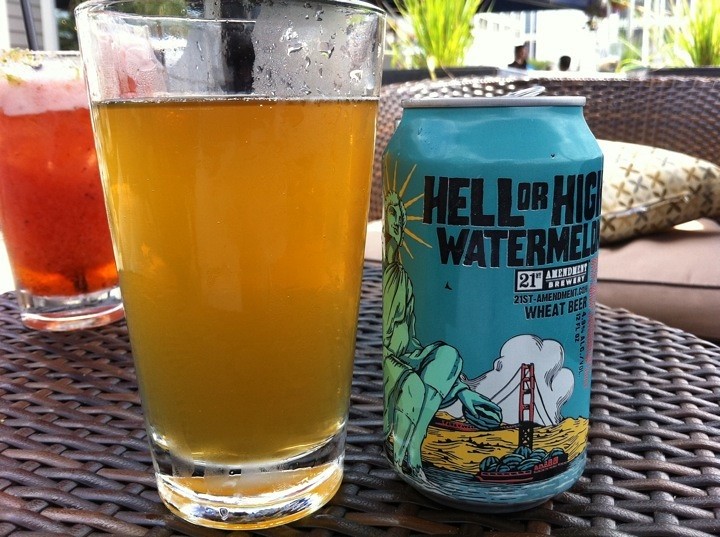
[379,96,603,510]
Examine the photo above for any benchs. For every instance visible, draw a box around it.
[363,73,720,340]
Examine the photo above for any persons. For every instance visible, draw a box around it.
[508,46,527,70]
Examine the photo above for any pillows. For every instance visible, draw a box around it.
[597,140,720,243]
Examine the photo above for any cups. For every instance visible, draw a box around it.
[0,52,125,334]
[73,0,389,529]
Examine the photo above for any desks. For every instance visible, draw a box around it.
[0,265,720,537]
